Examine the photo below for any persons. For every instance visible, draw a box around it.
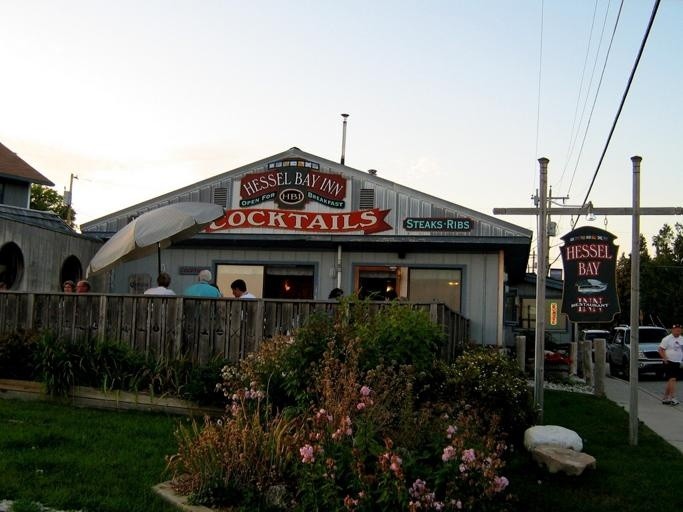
[63,280,74,292]
[181,270,223,298]
[328,288,344,300]
[658,324,683,405]
[143,270,177,298]
[231,279,255,299]
[358,289,398,302]
[77,281,90,293]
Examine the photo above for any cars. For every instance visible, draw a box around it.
[579,325,669,380]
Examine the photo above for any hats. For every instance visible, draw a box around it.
[673,324,682,328]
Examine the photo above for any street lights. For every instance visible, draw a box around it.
[67,173,78,225]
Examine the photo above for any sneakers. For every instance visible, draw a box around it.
[662,398,680,407]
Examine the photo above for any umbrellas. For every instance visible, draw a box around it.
[85,201,226,273]
